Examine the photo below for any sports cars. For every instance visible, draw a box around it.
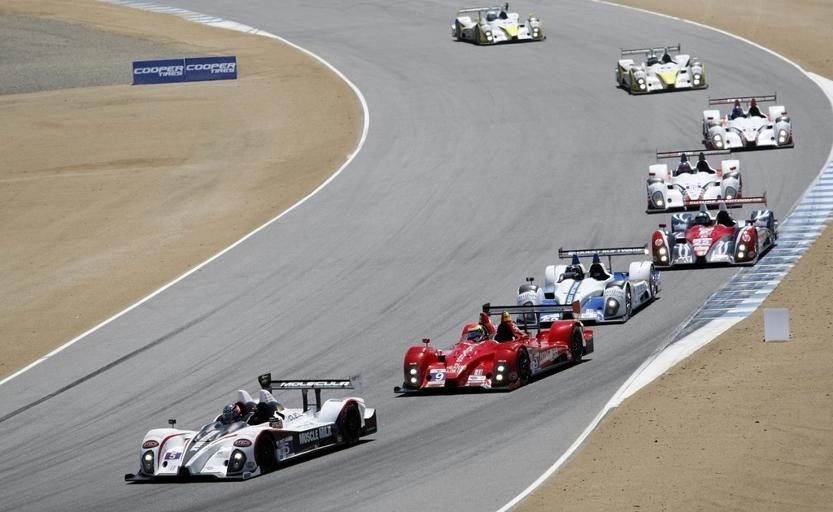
[451,1,547,45]
[124,371,378,482]
[512,243,661,325]
[647,147,742,214]
[394,299,595,395]
[701,93,794,152]
[651,192,778,269]
[615,44,708,95]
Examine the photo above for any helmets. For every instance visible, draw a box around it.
[648,55,658,65]
[732,107,743,118]
[564,265,580,280]
[486,11,497,21]
[695,212,710,224]
[223,403,241,423]
[468,325,488,342]
[678,162,691,174]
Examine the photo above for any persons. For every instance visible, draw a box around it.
[486,10,497,22]
[694,213,710,226]
[222,404,240,424]
[731,106,743,119]
[467,322,490,342]
[677,162,690,173]
[562,262,585,280]
[646,54,658,65]
